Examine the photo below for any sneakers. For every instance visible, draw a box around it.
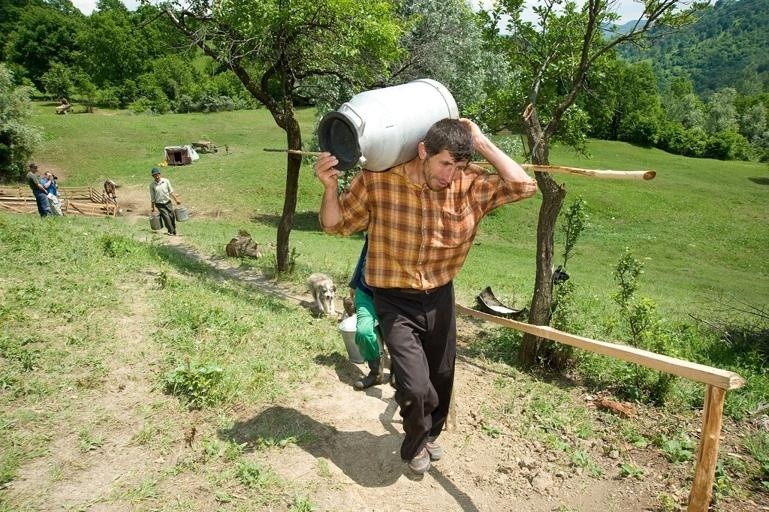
[427,441,443,460]
[406,448,430,475]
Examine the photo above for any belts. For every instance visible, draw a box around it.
[383,286,440,296]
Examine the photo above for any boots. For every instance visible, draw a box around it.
[390,361,396,387]
[354,358,385,388]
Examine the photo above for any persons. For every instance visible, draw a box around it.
[149,167,180,236]
[346,232,398,391]
[312,118,539,476]
[26,162,53,217]
[39,171,63,216]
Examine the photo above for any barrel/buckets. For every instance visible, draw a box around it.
[174,207,189,222]
[340,316,385,364]
[318,78,461,172]
[148,213,164,230]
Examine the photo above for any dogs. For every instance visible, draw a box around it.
[307,273,337,316]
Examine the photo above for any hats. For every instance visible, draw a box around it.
[29,163,39,167]
[151,168,160,176]
